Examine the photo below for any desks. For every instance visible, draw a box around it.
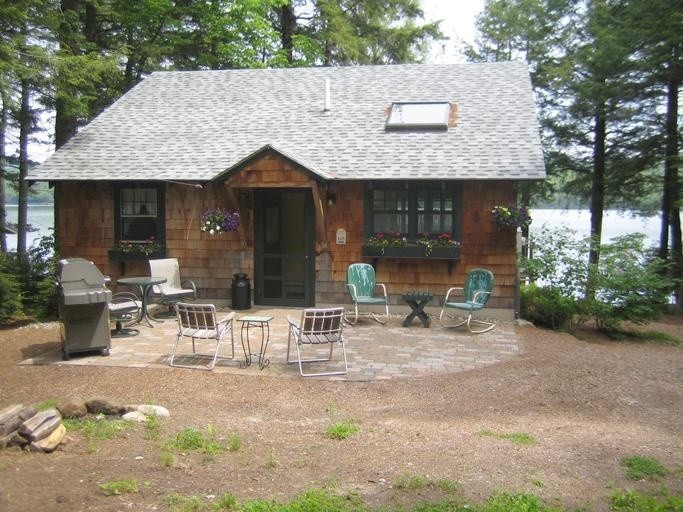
[402,291,433,327]
[237,316,275,371]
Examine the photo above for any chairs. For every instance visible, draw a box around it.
[286,308,349,376]
[439,268,495,333]
[104,276,142,337]
[170,302,236,371]
[148,258,196,318]
[344,263,389,326]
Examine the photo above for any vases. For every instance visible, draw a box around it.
[497,229,517,246]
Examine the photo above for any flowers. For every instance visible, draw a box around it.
[200,208,240,235]
[417,232,464,256]
[368,232,407,256]
[494,205,533,230]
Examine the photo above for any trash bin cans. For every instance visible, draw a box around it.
[230,273,252,311]
[54,258,113,361]
